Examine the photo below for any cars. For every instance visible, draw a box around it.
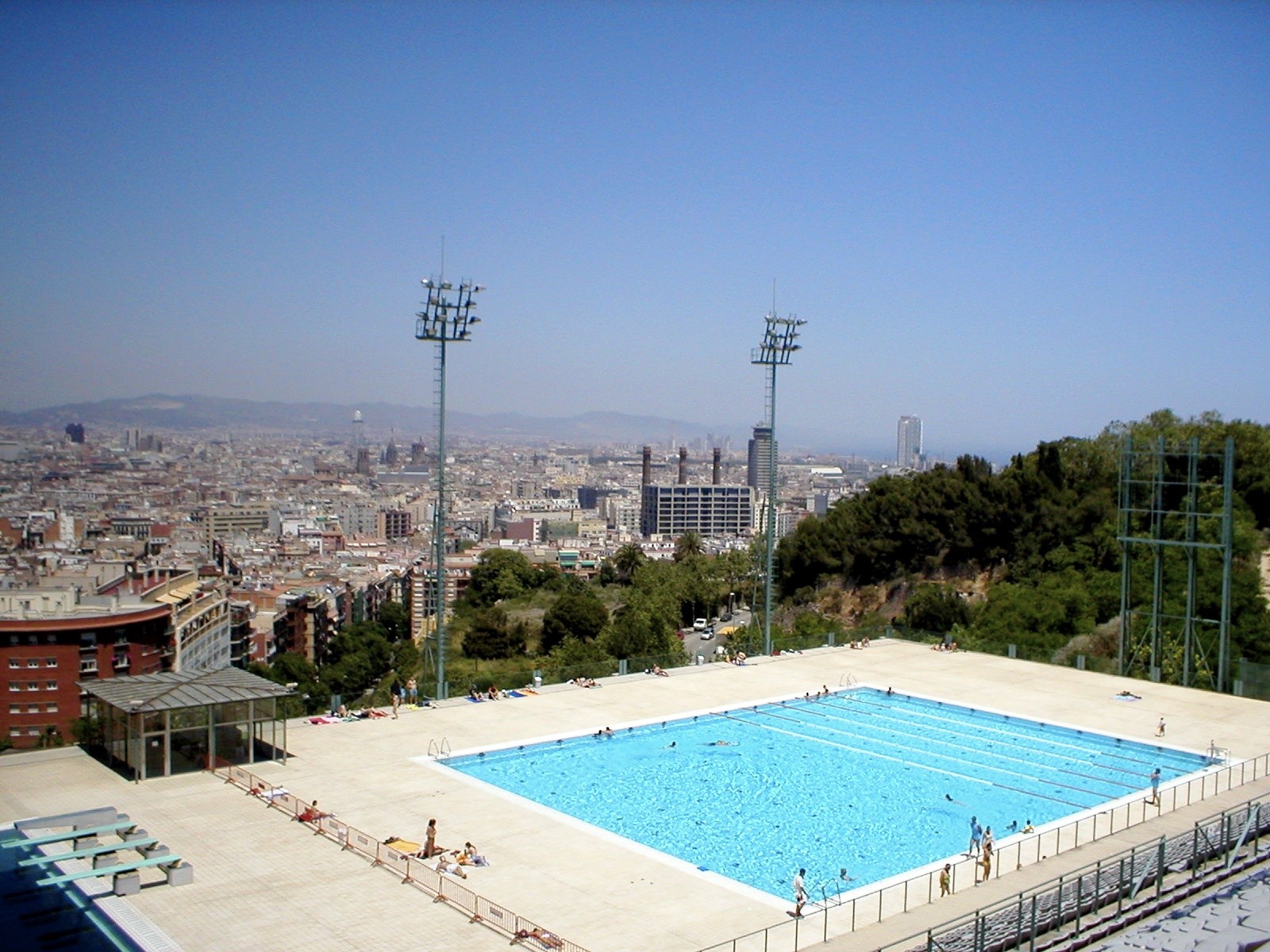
[720,612,732,622]
[700,628,715,640]
[675,631,684,641]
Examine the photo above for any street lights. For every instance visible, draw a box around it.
[411,276,487,701]
[748,312,808,657]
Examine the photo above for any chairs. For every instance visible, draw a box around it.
[901,802,1270,952]
[304,807,338,821]
[254,785,290,798]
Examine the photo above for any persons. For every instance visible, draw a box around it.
[664,741,677,749]
[887,687,894,694]
[804,684,829,698]
[1150,767,1162,807]
[514,928,564,949]
[601,726,615,737]
[467,683,514,703]
[725,652,747,666]
[709,740,731,745]
[325,701,388,720]
[1006,820,1035,834]
[311,801,332,817]
[652,664,669,677]
[1156,717,1166,736]
[841,868,858,881]
[849,634,871,648]
[524,685,540,694]
[938,815,995,897]
[770,648,797,656]
[391,677,432,720]
[1118,691,1142,699]
[793,868,810,919]
[576,676,596,688]
[932,640,957,652]
[425,819,477,880]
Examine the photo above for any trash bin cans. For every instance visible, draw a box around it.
[533,677,542,687]
[696,655,705,665]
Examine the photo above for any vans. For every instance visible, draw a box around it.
[693,617,708,632]
